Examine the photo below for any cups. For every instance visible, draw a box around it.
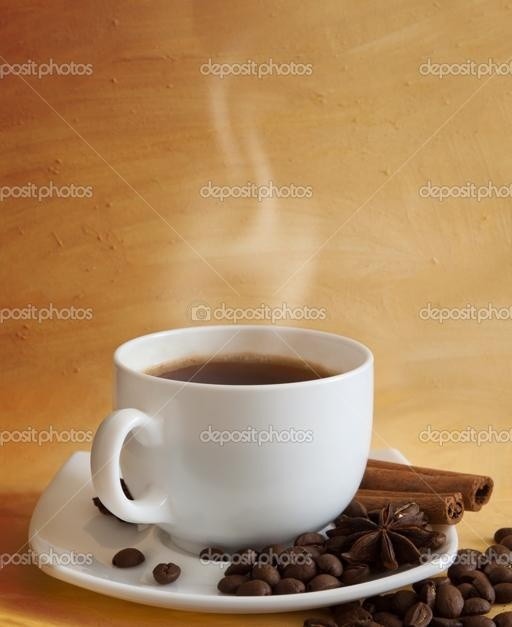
[91,324,376,559]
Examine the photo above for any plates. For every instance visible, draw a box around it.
[27,450,462,617]
